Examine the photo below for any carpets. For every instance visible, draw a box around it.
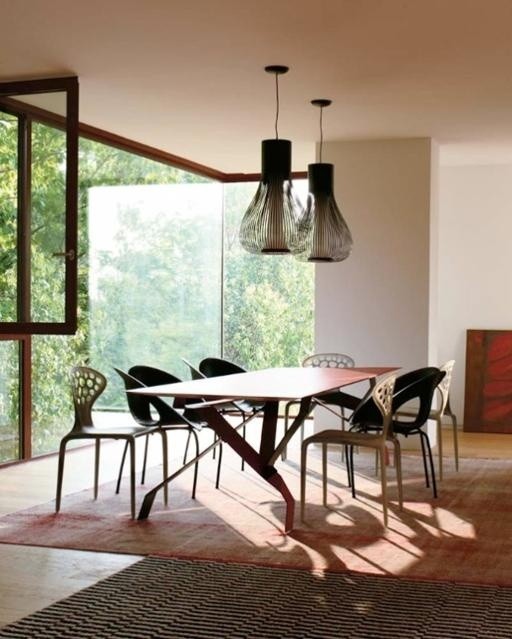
[0,446,512,587]
[0,557,512,638]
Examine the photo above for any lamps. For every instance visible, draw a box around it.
[291,98,353,263]
[240,65,313,256]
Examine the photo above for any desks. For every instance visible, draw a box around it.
[120,365,403,535]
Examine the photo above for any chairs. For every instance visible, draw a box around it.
[344,366,448,501]
[296,370,398,527]
[112,361,228,503]
[179,355,266,473]
[53,366,168,519]
[277,353,357,458]
[394,358,458,484]
